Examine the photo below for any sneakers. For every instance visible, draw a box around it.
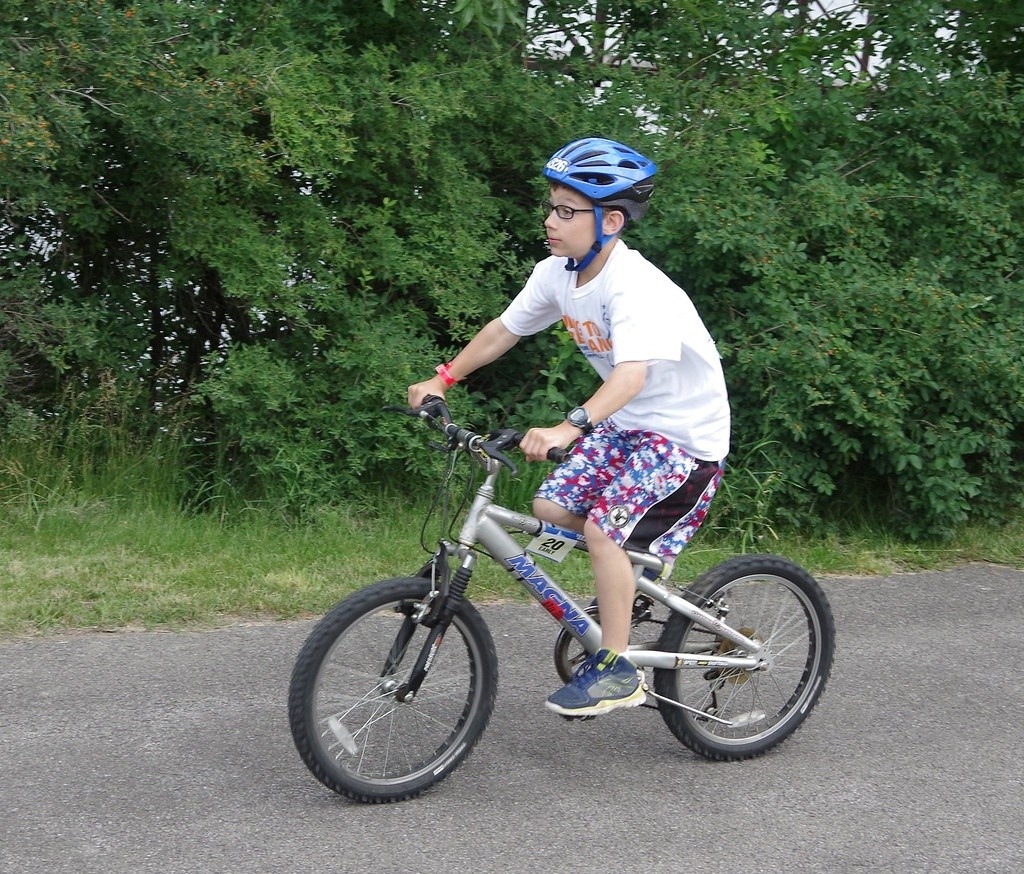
[545,648,647,717]
[588,552,672,609]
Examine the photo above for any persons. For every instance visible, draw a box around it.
[406,135,732,718]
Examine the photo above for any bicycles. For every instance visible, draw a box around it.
[289,396,837,804]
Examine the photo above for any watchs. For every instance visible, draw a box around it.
[566,406,593,435]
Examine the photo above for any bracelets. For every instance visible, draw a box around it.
[435,362,454,387]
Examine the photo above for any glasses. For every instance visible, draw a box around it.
[540,201,595,219]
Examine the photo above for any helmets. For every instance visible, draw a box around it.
[542,136,657,221]
